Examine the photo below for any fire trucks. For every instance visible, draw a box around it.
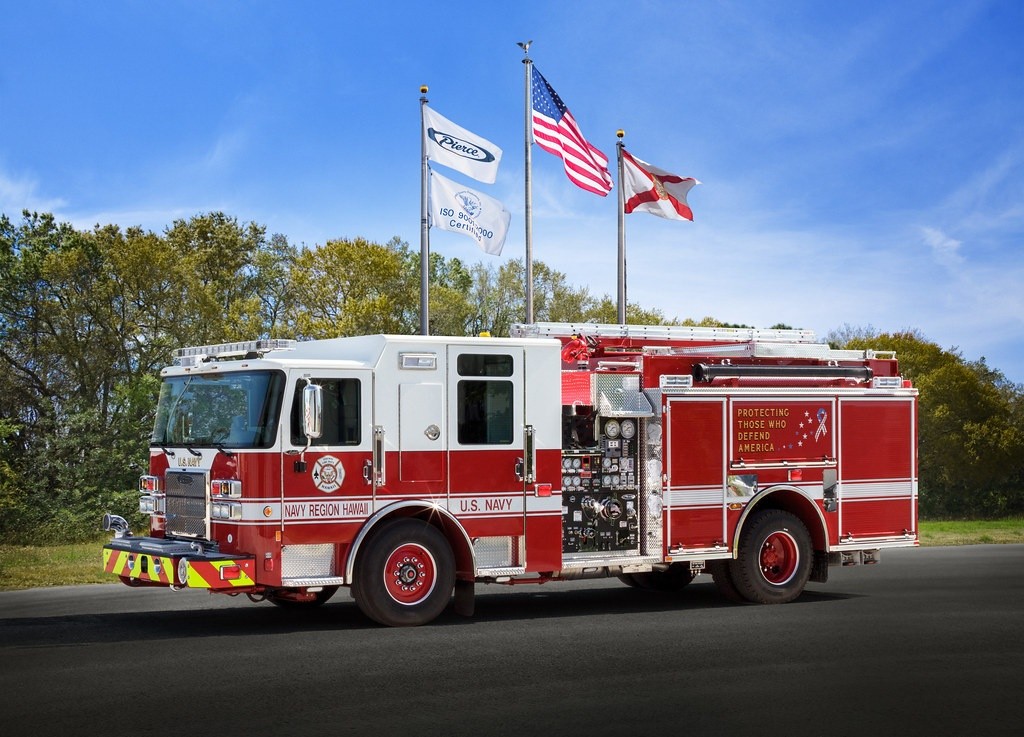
[102,321,919,626]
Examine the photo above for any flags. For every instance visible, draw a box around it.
[430,169,511,256]
[423,104,503,185]
[531,64,615,197]
[621,147,702,222]
